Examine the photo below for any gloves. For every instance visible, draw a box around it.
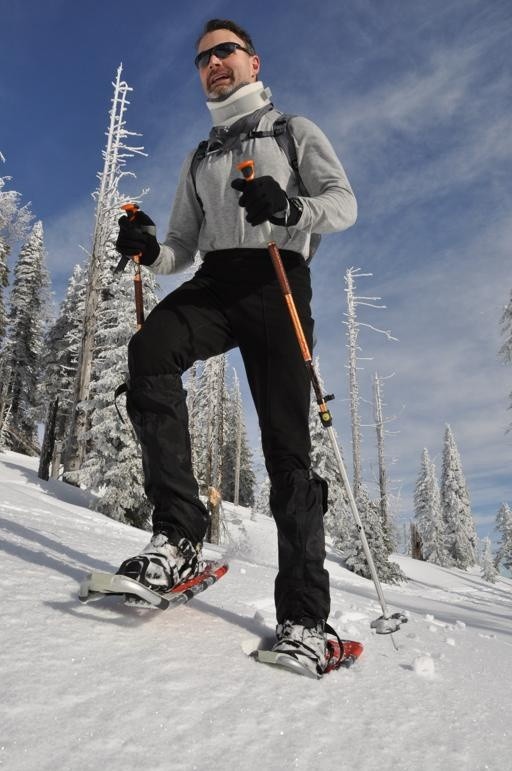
[111,209,161,267]
[232,175,289,227]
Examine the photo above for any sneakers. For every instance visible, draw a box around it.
[270,617,328,675]
[114,529,201,590]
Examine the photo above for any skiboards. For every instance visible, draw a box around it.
[79,560,364,680]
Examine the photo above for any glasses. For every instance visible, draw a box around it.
[193,41,253,70]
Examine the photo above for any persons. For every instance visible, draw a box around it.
[116,17,360,673]
